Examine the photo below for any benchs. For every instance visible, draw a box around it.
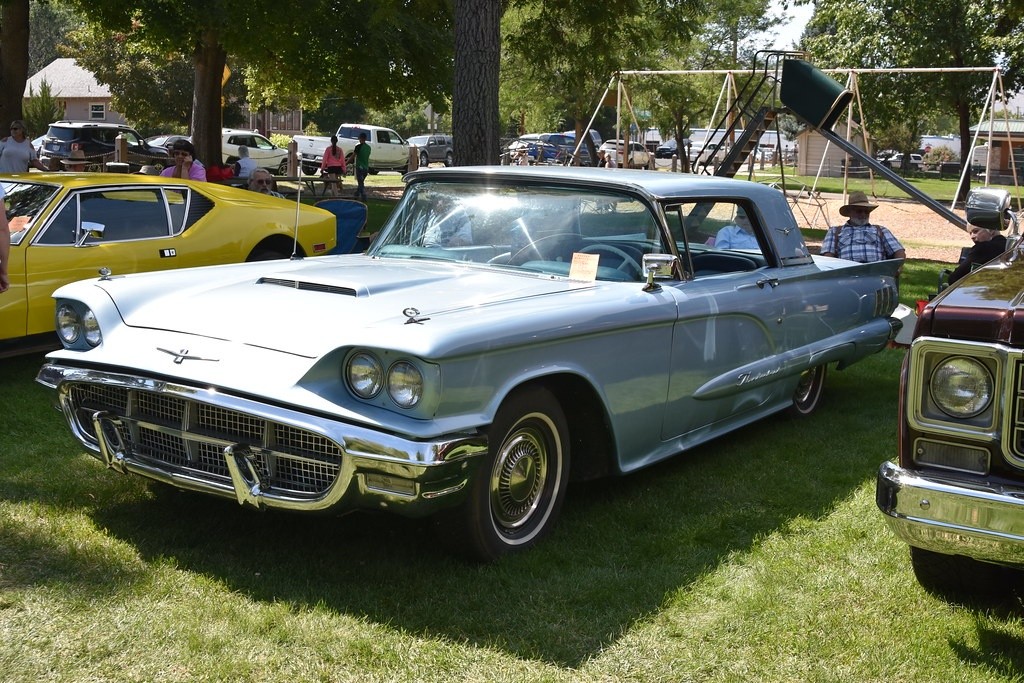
[977,168,1021,185]
[584,238,766,268]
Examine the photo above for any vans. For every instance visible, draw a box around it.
[406,134,453,166]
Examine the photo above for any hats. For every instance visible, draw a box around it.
[134,165,156,175]
[839,191,879,216]
[60,150,92,165]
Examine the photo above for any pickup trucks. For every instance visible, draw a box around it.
[292,123,420,176]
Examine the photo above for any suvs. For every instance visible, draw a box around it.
[878,153,925,171]
[188,128,302,176]
[30,118,187,172]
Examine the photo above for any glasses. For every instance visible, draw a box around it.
[10,127,18,131]
[851,208,869,215]
[738,214,746,220]
[257,179,272,186]
[174,151,188,157]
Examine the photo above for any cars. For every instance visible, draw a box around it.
[655,137,694,155]
[507,129,602,166]
[34,166,904,563]
[0,172,336,358]
[875,186,1024,589]
[598,139,654,169]
[684,141,775,166]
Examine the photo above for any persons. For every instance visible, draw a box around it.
[346,132,371,203]
[321,135,347,196]
[941,221,1007,285]
[245,168,287,200]
[0,120,48,175]
[820,190,906,276]
[0,198,10,295]
[784,144,798,156]
[159,140,208,182]
[715,205,761,250]
[60,150,94,171]
[232,146,257,176]
[48,157,63,171]
[515,146,529,165]
[596,150,615,168]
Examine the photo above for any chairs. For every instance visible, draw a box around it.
[314,198,368,255]
